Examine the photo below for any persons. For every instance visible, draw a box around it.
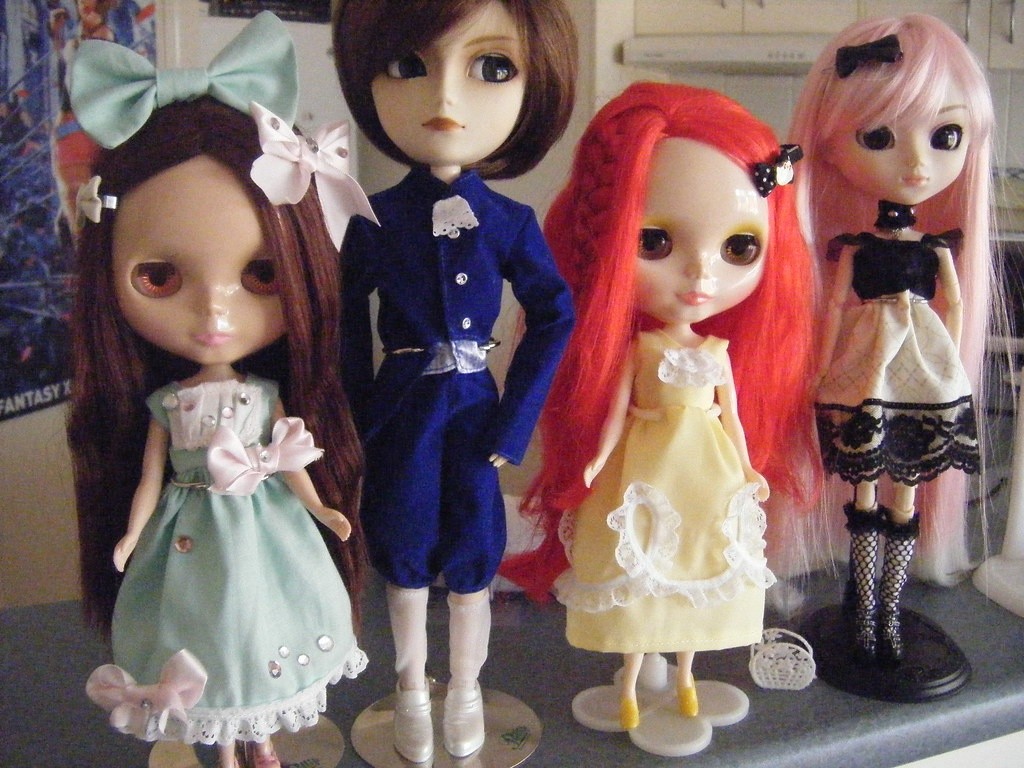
[790,12,995,661]
[332,1,579,764]
[499,83,812,729]
[70,8,379,768]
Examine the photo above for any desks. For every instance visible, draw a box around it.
[1,471,1024,766]
